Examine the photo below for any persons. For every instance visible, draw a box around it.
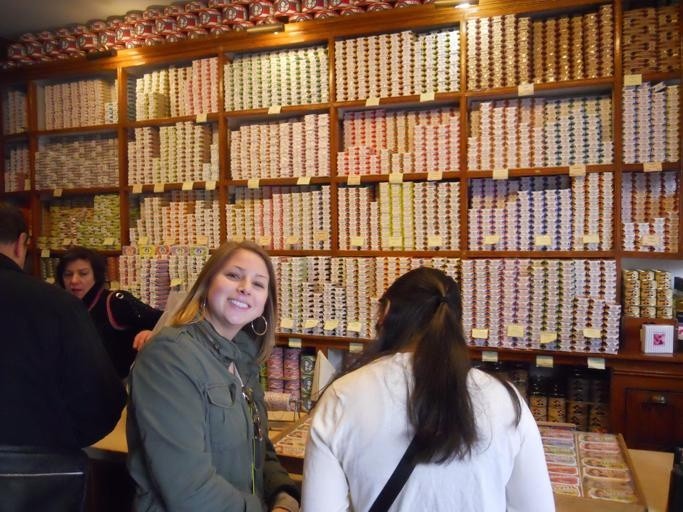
[125,241,300,511]
[294,267,553,511]
[42,246,163,452]
[0,200,121,511]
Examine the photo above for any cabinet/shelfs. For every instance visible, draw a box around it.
[224,18,336,357]
[333,1,469,357]
[30,49,123,296]
[463,0,620,370]
[1,62,40,275]
[621,2,681,361]
[610,352,681,452]
[122,34,223,311]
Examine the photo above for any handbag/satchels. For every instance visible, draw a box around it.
[0,444,87,512]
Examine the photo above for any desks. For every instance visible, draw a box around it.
[82,405,676,511]
